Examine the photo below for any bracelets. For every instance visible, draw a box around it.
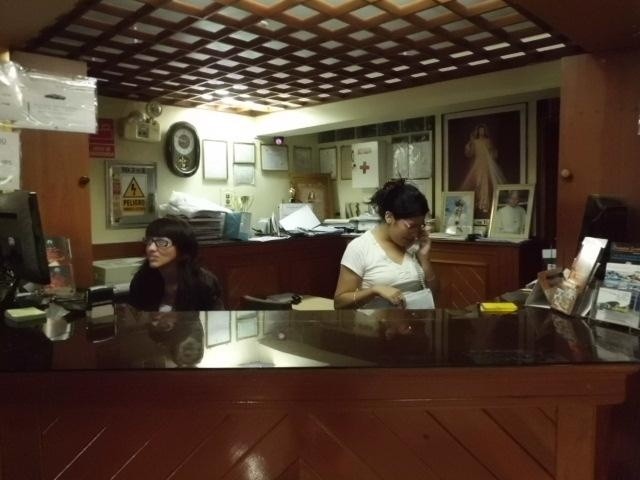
[353,291,359,309]
[425,274,436,283]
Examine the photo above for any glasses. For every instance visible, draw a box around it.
[143,237,172,248]
[404,221,426,229]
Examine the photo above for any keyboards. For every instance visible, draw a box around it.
[53,295,88,311]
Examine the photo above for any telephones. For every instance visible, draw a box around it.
[406,237,420,254]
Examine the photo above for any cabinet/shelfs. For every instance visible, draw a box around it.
[197,235,338,310]
[340,234,540,310]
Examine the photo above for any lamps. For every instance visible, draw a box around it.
[273,136,284,145]
[119,101,163,143]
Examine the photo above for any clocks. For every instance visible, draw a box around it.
[165,122,200,177]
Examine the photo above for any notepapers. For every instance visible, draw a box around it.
[5,306,47,323]
[480,302,518,312]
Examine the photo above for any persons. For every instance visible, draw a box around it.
[128,216,225,311]
[457,124,506,212]
[492,191,526,235]
[334,174,437,312]
[447,198,468,235]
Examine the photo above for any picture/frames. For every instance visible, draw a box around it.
[293,147,312,171]
[260,144,289,172]
[488,184,535,239]
[442,191,475,236]
[202,139,228,181]
[319,147,338,181]
[341,145,352,180]
[440,102,528,226]
[233,142,256,164]
[289,173,334,224]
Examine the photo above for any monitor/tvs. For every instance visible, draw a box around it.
[0,191,51,313]
[578,194,640,280]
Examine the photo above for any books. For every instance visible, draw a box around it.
[187,191,233,241]
